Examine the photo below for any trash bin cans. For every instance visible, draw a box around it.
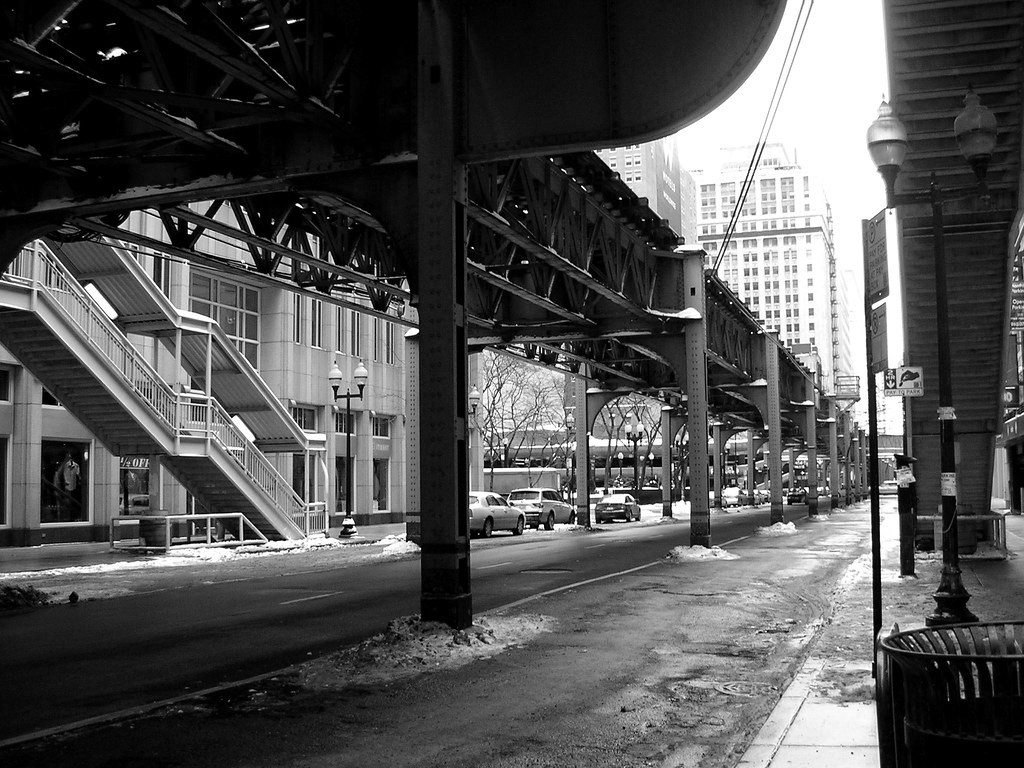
[139,509,170,551]
[880,619,1024,768]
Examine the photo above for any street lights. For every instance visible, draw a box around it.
[648,452,654,483]
[625,421,644,503]
[675,433,690,506]
[328,359,369,539]
[617,451,623,478]
[503,437,508,470]
[722,441,731,489]
[865,80,1000,627]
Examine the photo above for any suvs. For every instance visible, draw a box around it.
[507,488,576,531]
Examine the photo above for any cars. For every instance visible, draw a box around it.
[709,487,771,509]
[595,493,642,524]
[469,491,527,539]
[787,487,810,505]
